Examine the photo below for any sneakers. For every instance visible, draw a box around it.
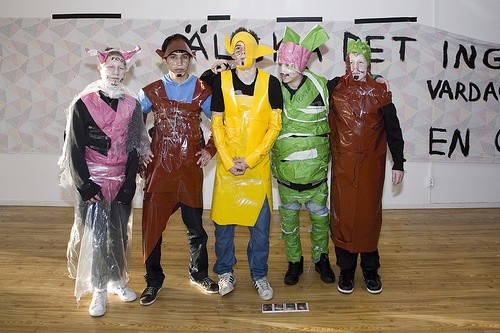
[364,273,383,293]
[337,269,355,293]
[252,276,273,300]
[139,286,162,305]
[107,284,137,302]
[190,276,218,294]
[316,252,335,282]
[217,271,236,295]
[284,256,304,284]
[88,290,107,316]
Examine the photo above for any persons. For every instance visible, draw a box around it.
[60,47,147,317]
[211,28,285,300]
[327,31,405,294]
[212,39,390,286]
[136,33,220,305]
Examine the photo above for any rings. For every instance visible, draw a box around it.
[202,157,205,161]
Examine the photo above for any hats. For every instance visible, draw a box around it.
[155,38,198,58]
[278,25,329,71]
[347,38,371,63]
